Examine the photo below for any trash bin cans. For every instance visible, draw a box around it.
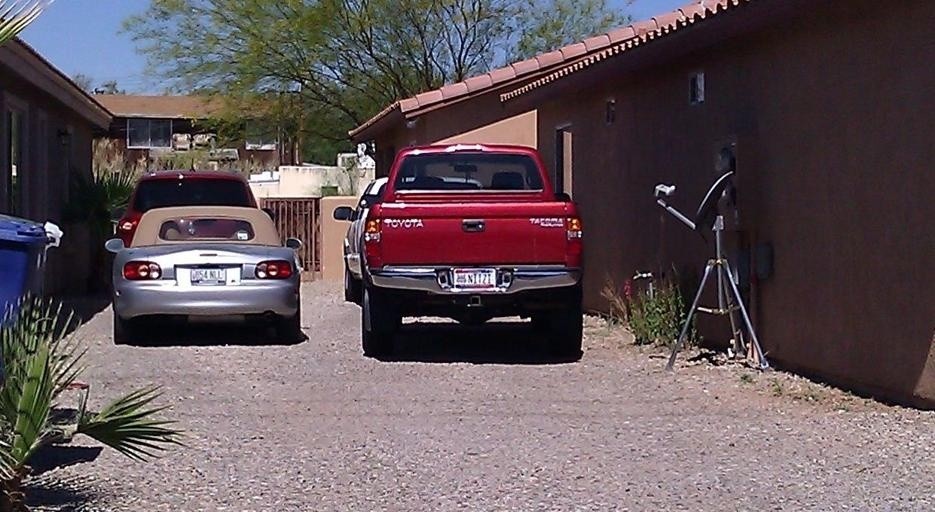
[0,215,56,327]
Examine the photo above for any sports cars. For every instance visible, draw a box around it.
[104,204,303,346]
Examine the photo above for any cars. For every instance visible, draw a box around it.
[114,156,260,249]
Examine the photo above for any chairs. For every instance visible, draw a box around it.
[408,170,524,189]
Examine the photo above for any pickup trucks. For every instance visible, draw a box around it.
[357,141,586,358]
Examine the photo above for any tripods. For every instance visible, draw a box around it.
[664,218,769,379]
